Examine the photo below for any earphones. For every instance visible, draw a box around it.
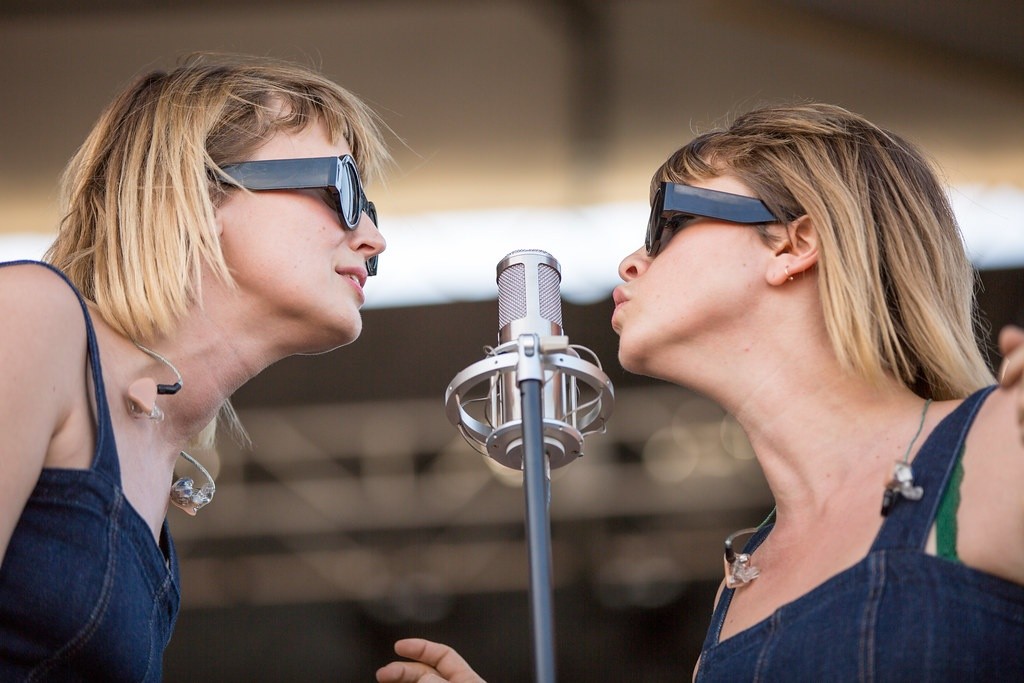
[124,377,164,421]
[169,477,214,517]
[882,460,924,514]
[722,541,759,588]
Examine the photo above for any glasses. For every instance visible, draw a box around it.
[644,179,795,258]
[208,154,379,277]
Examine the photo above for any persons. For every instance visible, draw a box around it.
[374,103,1024,683]
[0,52,385,683]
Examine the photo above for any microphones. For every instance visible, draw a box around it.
[443,248,615,514]
